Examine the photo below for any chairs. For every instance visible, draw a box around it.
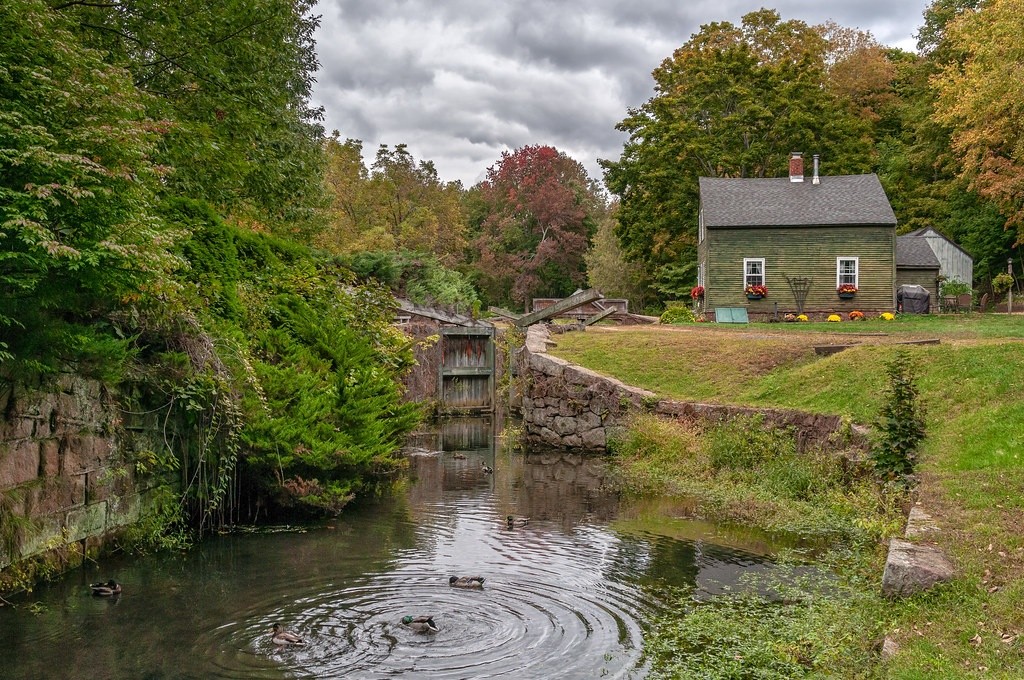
[955,293,972,314]
[945,295,960,314]
[973,293,988,313]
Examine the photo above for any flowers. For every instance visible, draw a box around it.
[746,285,767,297]
[691,286,704,297]
[839,285,856,293]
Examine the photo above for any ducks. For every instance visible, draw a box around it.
[449,573,486,588]
[506,514,531,524]
[403,614,437,633]
[89,579,123,598]
[453,451,493,474]
[267,621,307,647]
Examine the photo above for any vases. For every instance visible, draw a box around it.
[840,292,854,297]
[746,294,762,298]
[697,296,704,300]
